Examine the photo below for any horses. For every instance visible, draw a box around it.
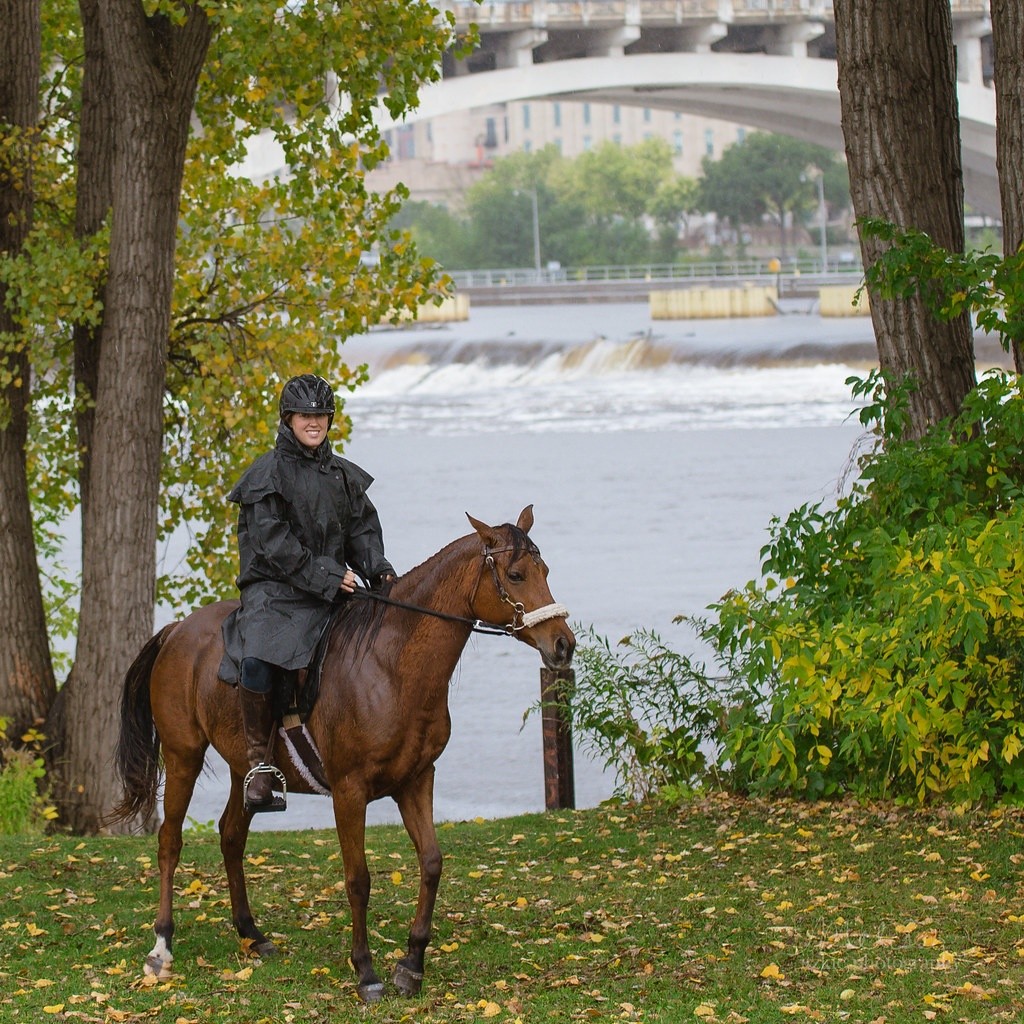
[89,499,576,1002]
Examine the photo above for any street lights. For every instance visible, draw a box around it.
[800,170,827,275]
[514,189,541,283]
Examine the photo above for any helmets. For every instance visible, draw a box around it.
[279,374,335,432]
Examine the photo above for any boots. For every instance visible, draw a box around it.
[239,676,288,807]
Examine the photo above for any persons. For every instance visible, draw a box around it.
[214,372,397,809]
